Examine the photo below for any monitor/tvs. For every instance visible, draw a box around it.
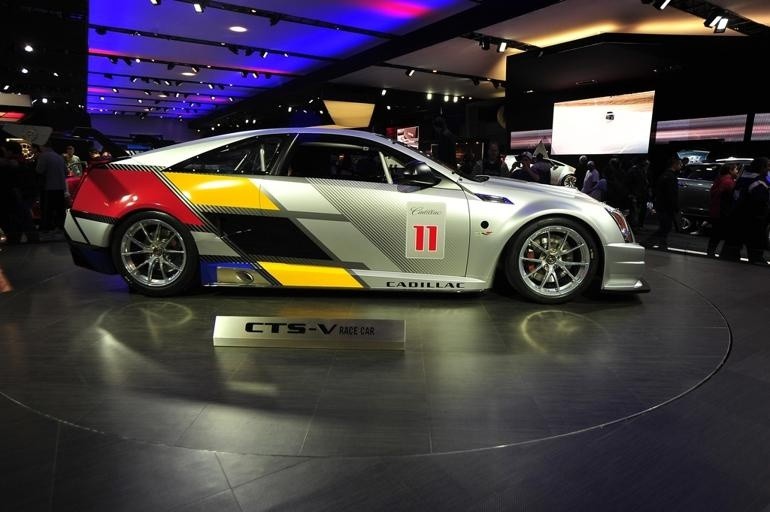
[395,125,420,150]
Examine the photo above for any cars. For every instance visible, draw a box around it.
[497,145,767,235]
[59,125,656,309]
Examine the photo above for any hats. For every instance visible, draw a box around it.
[522,152,532,161]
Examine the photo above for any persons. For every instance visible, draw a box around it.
[0,141,112,245]
